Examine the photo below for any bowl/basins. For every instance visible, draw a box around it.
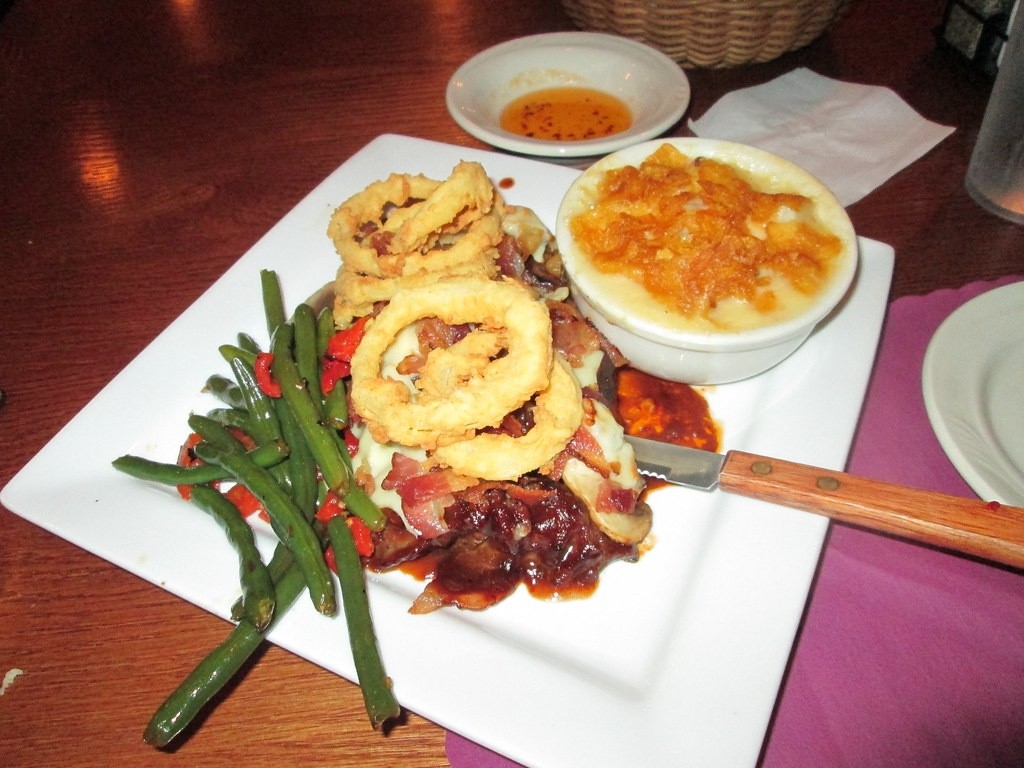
[556,135,861,389]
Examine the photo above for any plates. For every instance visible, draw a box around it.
[921,281,1024,509]
[445,30,692,169]
[2,130,895,768]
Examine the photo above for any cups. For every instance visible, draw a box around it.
[964,0,1024,227]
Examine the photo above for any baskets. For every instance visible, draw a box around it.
[562,0,845,69]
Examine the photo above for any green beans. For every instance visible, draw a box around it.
[110,269,400,746]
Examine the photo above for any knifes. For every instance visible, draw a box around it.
[622,433,1024,576]
